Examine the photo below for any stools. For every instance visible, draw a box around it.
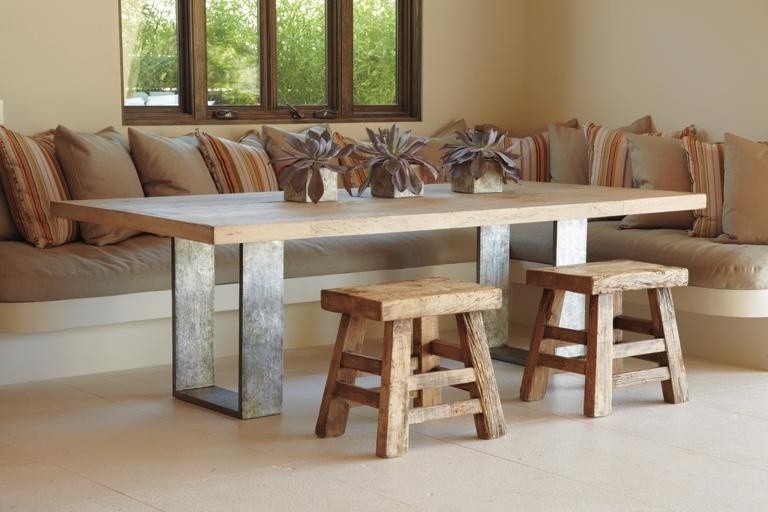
[315,277,507,458]
[519,259,689,418]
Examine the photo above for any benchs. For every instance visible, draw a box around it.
[0,225,480,388]
[509,220,768,369]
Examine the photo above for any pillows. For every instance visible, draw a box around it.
[475,113,768,244]
